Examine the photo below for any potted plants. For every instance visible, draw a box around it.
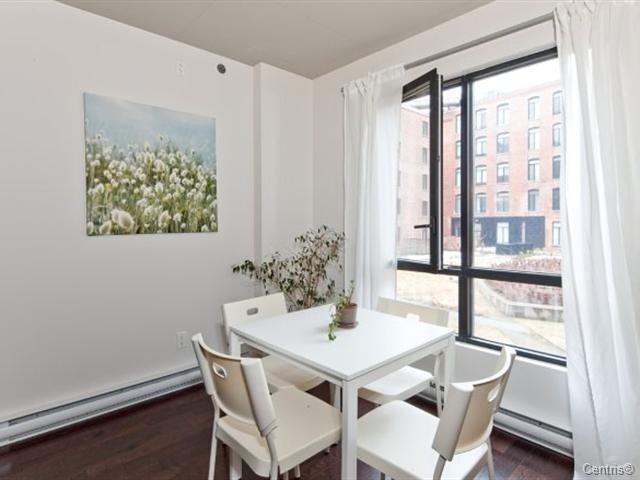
[325,279,361,339]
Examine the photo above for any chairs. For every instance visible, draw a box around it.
[189,293,518,480]
